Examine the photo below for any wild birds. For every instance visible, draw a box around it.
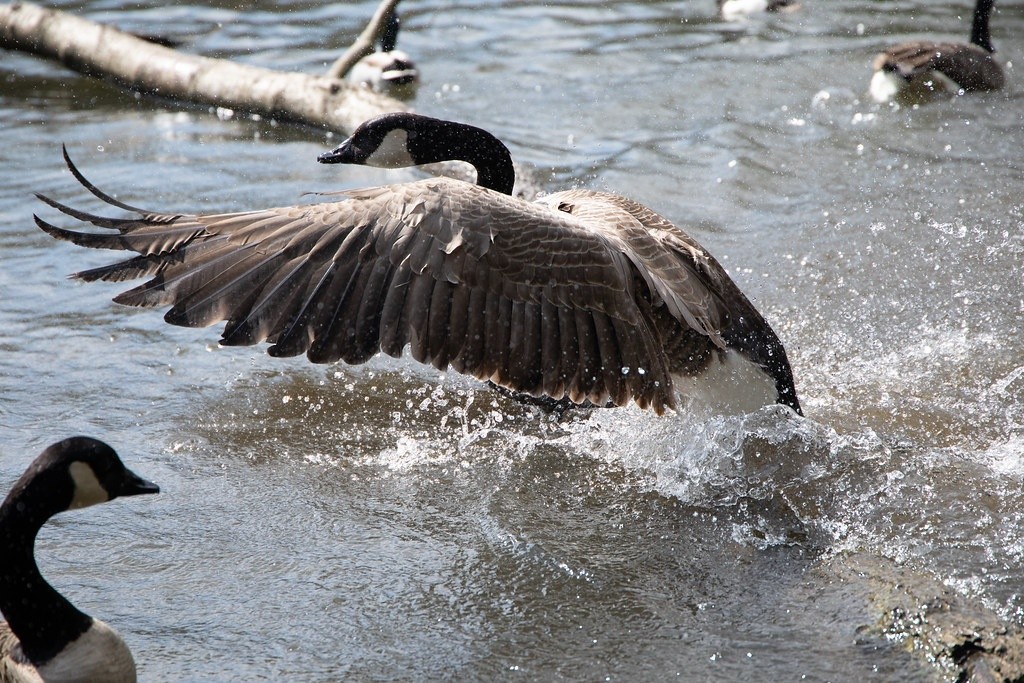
[353,8,421,99]
[873,0,1005,102]
[33,112,807,421]
[0,435,163,682]
